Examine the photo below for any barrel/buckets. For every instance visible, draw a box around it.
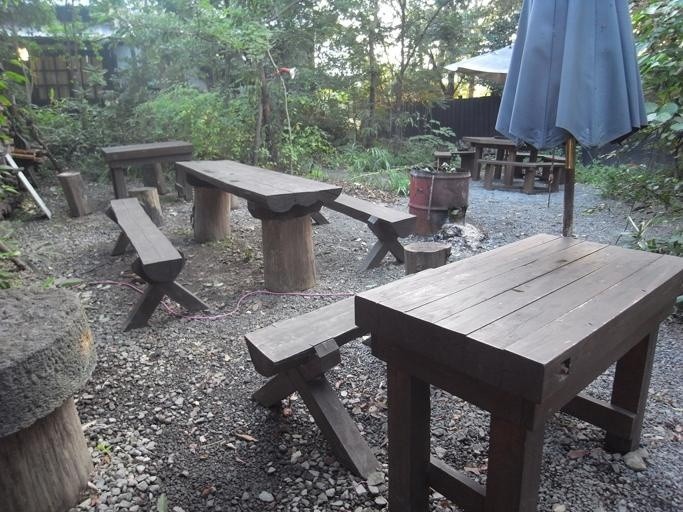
[409,164,470,236]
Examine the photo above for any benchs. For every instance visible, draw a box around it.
[312,195,414,271]
[105,197,209,330]
[434,151,566,194]
[244,295,380,480]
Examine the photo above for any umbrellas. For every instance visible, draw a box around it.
[495,1,647,237]
[443,43,515,87]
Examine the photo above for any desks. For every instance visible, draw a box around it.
[463,137,537,180]
[0,289,99,512]
[355,233,683,512]
[101,141,194,201]
[175,159,342,292]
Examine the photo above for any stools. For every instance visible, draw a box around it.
[404,241,452,276]
[58,172,93,217]
[129,187,163,227]
